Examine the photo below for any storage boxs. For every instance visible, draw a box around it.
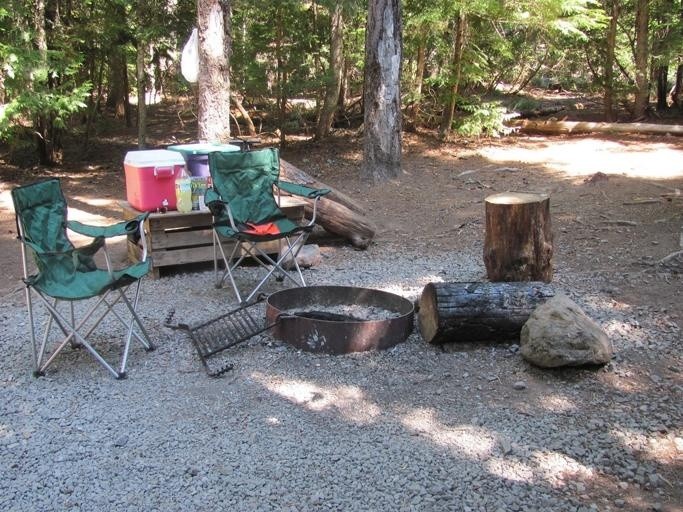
[124,149,186,214]
[167,142,240,178]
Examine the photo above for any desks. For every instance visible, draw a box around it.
[119,195,308,280]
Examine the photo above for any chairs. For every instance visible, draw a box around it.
[10,177,155,380]
[204,147,330,307]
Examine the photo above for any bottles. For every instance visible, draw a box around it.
[191,176,206,211]
[175,165,193,213]
